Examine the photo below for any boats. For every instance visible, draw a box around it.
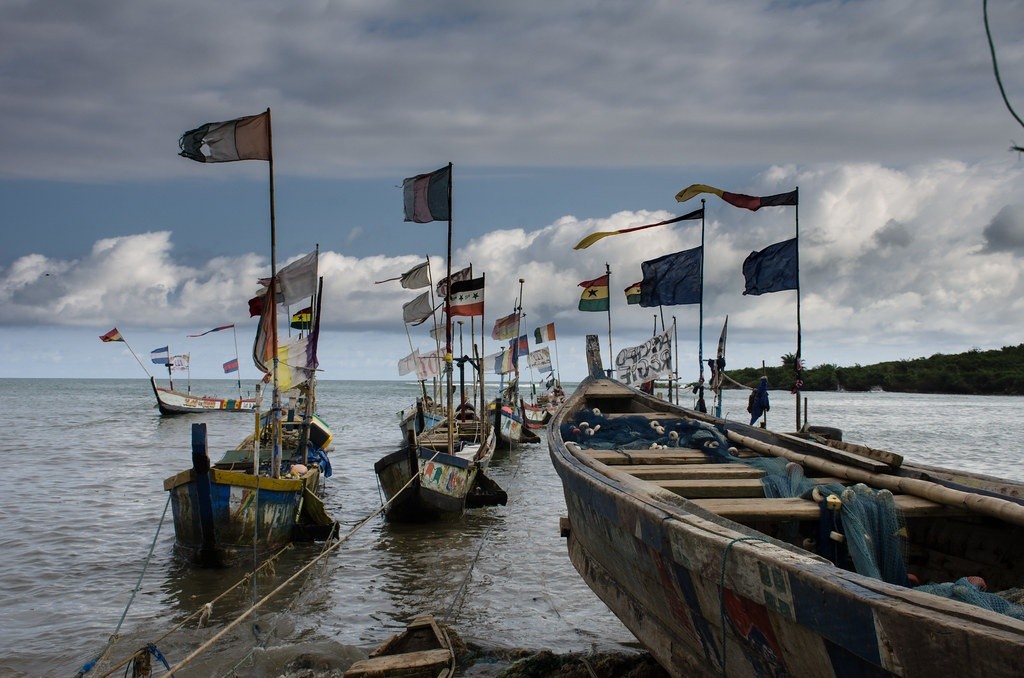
[150,362,261,414]
[546,333,1023,678]
[165,276,333,569]
[373,277,565,520]
[345,615,455,678]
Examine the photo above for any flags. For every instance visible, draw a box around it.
[484,313,552,374]
[249,250,319,393]
[624,282,641,305]
[171,355,189,371]
[445,277,484,317]
[402,165,449,223]
[178,112,269,163]
[791,358,804,394]
[534,322,555,344]
[151,346,169,364]
[615,326,673,388]
[640,246,701,307]
[742,238,797,296]
[436,267,470,297]
[398,321,453,378]
[402,292,433,323]
[577,275,610,312]
[750,380,770,426]
[223,358,238,374]
[400,261,430,289]
[99,328,125,342]
